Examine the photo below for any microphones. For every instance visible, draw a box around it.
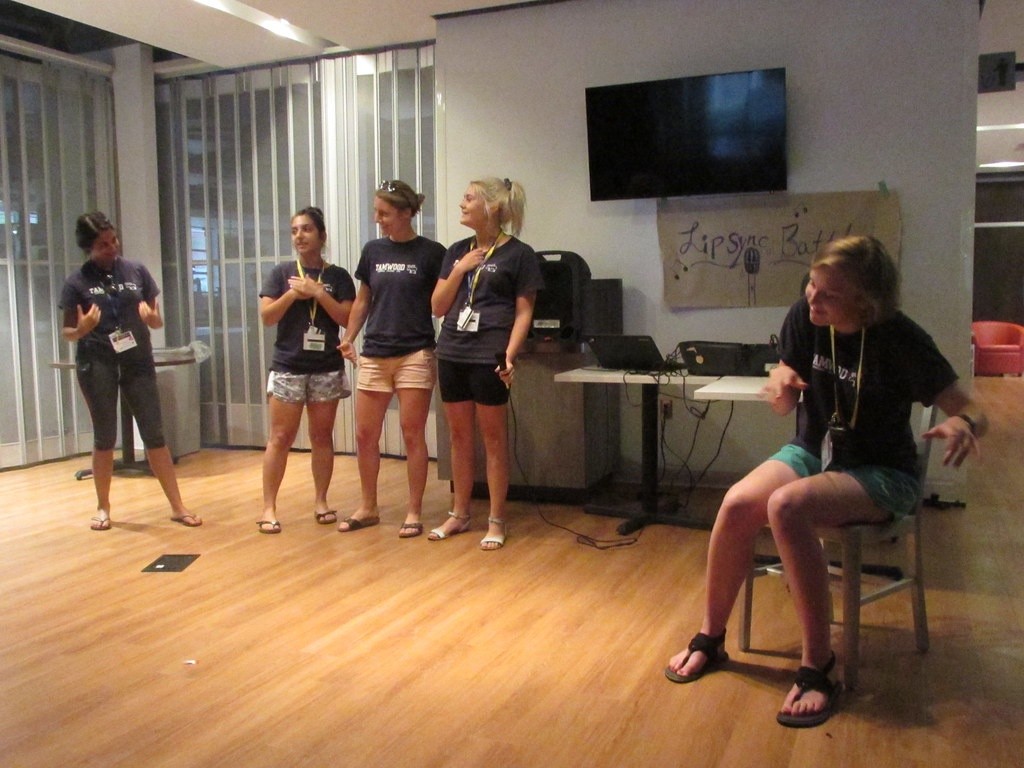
[495,350,510,390]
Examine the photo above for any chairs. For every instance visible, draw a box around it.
[737,397,935,697]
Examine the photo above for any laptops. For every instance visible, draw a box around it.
[582,335,686,371]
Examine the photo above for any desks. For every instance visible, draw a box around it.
[48,350,196,479]
[554,370,904,580]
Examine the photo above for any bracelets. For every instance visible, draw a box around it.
[954,413,977,434]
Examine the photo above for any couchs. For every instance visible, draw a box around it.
[971,321,1024,375]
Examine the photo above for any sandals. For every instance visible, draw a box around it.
[428,511,472,540]
[480,517,508,550]
[665,628,729,682]
[776,650,843,728]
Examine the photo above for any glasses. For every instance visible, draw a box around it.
[104,274,119,296]
[380,180,411,206]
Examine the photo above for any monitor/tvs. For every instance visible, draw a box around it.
[585,66,788,201]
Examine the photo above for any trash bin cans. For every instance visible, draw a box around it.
[152,349,201,459]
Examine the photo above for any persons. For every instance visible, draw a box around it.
[58,211,203,532]
[255,206,357,535]
[430,176,539,551]
[663,235,984,729]
[337,180,447,539]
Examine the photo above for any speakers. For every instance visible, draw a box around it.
[525,250,592,344]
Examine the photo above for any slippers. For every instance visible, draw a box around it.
[90,517,111,530]
[256,520,281,534]
[338,516,380,532]
[313,510,337,524]
[170,514,203,527]
[399,522,423,538]
